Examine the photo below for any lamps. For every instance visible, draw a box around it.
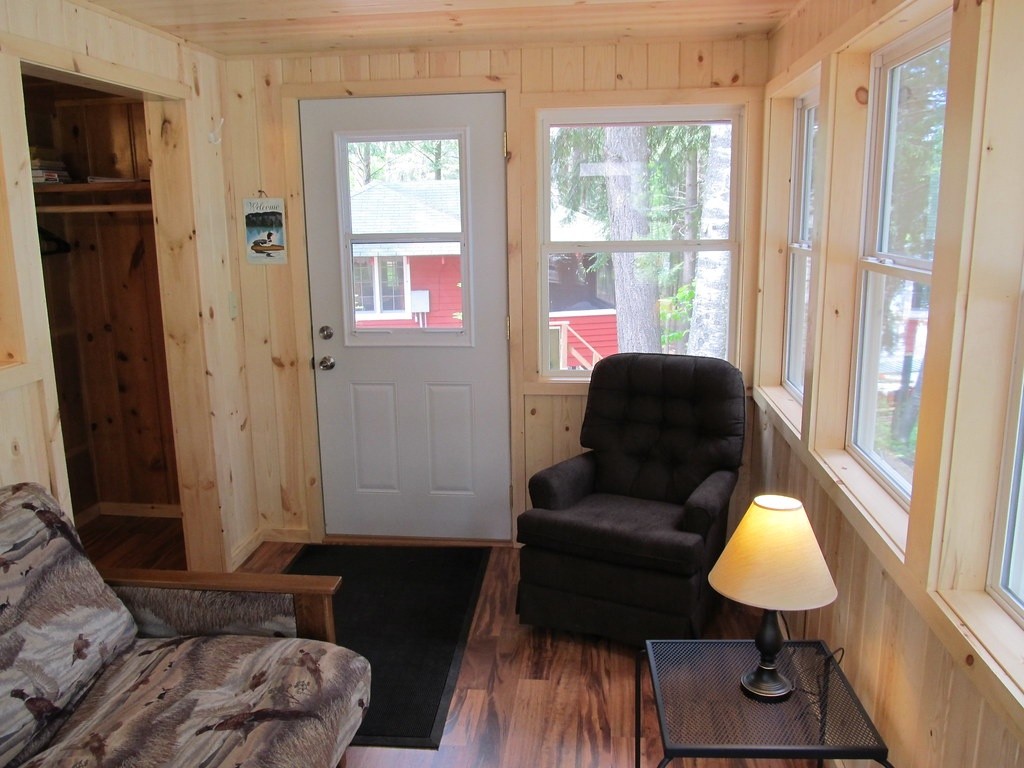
[706,494,839,701]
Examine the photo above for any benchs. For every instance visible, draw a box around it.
[0,483,372,768]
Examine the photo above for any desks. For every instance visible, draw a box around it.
[634,639,896,768]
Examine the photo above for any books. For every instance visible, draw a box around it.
[88,176,136,183]
[30,148,72,183]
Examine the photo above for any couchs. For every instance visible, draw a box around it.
[516,352,748,656]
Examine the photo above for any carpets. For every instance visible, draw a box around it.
[279,544,493,750]
